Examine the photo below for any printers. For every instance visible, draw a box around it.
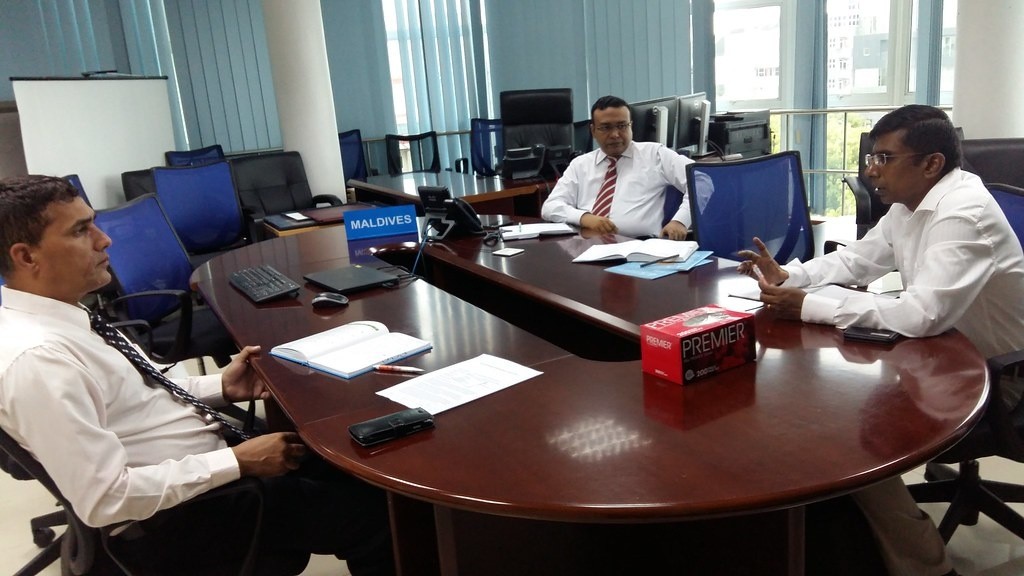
[708,110,771,159]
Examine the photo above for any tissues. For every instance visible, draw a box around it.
[640,303,756,385]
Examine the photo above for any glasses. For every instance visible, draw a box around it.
[865,153,923,167]
[594,121,632,133]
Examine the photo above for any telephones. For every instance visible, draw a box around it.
[417,185,482,234]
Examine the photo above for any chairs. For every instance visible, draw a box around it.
[0,401,312,576]
[663,126,1024,547]
[63,88,575,365]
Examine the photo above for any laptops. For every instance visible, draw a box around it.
[303,264,400,294]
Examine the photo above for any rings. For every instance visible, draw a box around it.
[768,304,770,308]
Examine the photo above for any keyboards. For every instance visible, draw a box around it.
[229,265,300,303]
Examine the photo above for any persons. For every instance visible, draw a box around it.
[736,104,1024,449]
[0,173,397,576]
[541,95,714,242]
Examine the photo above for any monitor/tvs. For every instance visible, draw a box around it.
[626,92,715,158]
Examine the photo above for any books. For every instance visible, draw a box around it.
[262,212,315,230]
[499,222,578,241]
[572,238,699,262]
[268,320,433,380]
[843,328,899,344]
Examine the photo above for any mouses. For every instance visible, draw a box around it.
[311,292,349,308]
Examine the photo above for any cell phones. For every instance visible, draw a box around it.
[483,220,514,230]
[348,407,434,447]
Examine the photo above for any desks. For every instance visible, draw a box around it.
[262,221,344,238]
[347,156,751,214]
[190,215,990,576]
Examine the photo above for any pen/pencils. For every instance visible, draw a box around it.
[640,254,679,268]
[372,365,426,372]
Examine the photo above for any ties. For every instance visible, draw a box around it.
[84,308,250,441]
[591,156,621,218]
